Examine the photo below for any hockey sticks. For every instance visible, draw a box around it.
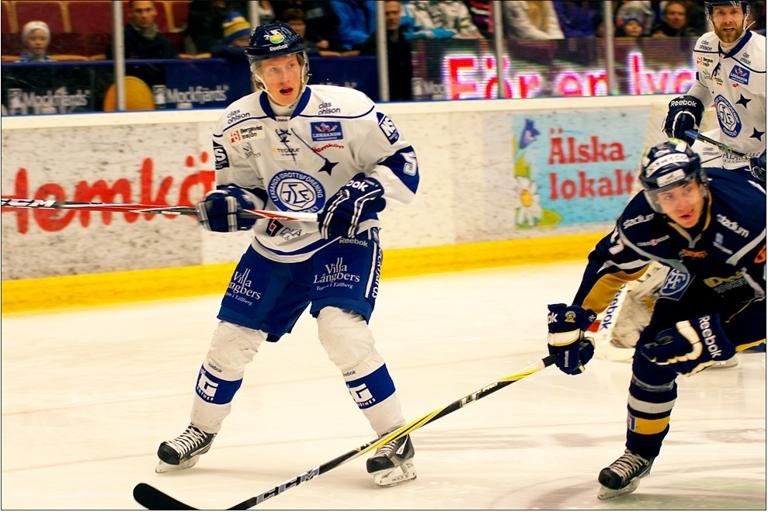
[134,337,597,510]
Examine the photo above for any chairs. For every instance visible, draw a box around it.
[1,0,193,62]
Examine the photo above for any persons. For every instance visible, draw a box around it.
[594,1,766,374]
[103,2,177,60]
[185,1,765,104]
[540,135,767,491]
[12,21,59,62]
[151,18,421,476]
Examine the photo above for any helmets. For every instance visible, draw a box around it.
[639,138,707,214]
[243,20,307,61]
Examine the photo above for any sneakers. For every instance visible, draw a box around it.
[367,432,414,473]
[158,423,217,464]
[598,449,653,489]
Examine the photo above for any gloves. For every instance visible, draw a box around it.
[319,173,384,240]
[664,95,704,147]
[197,187,256,232]
[547,304,597,375]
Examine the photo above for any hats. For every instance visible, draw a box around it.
[222,11,252,43]
